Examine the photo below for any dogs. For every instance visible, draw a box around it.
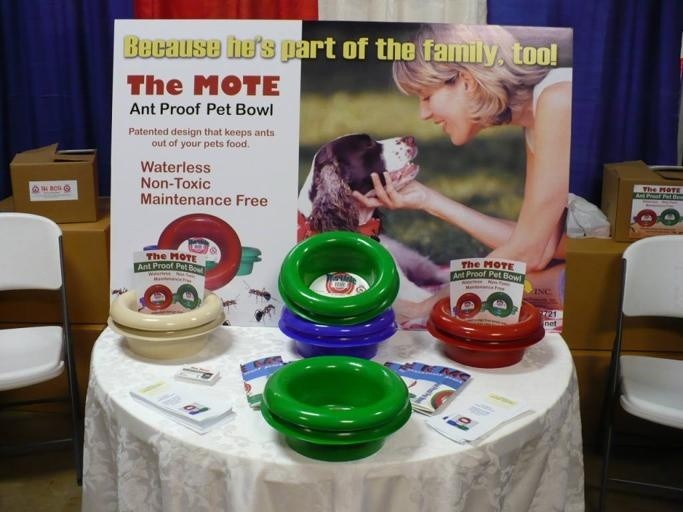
[297,133,420,245]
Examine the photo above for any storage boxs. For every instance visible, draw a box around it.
[0,142,108,408]
[563,160,683,448]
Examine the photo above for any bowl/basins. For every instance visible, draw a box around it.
[260,361,413,463]
[277,232,401,361]
[109,285,226,358]
[426,297,544,367]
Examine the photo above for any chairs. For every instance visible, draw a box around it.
[0,211,85,485]
[594,234,683,512]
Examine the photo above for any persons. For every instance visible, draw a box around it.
[351,22,574,320]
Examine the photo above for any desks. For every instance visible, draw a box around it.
[89,323,574,512]
